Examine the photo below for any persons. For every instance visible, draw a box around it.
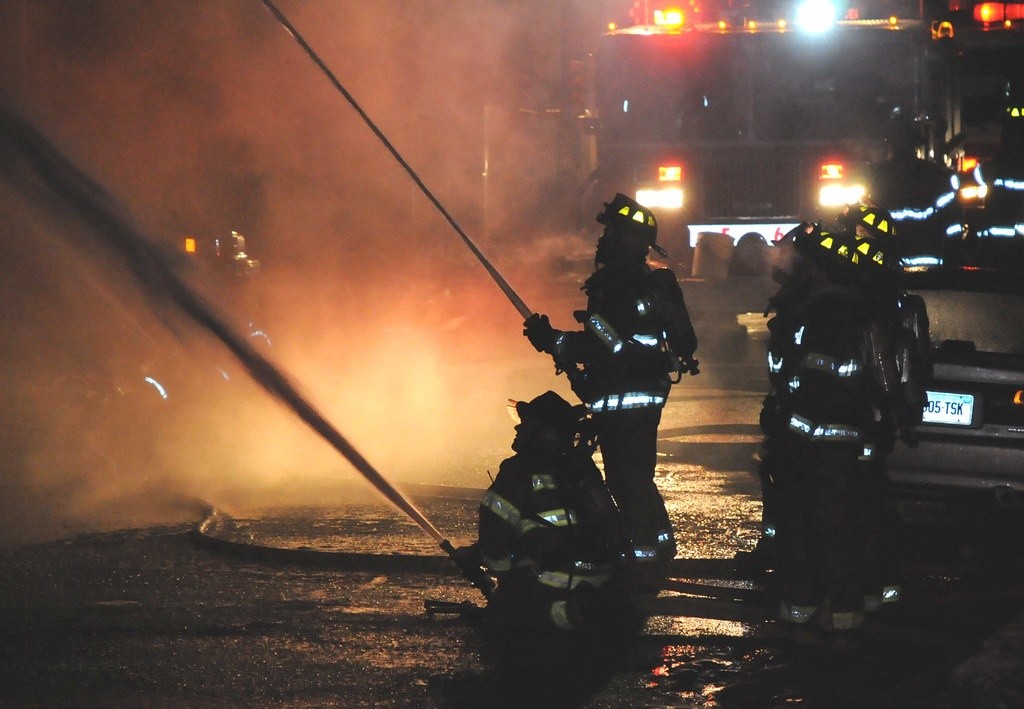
[736,204,931,653]
[448,390,635,697]
[529,193,678,571]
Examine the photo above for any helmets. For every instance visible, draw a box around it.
[597,193,658,241]
[855,205,896,237]
[516,392,578,445]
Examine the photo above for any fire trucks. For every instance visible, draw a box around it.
[563,1,925,322]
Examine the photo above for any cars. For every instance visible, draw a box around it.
[882,285,1024,527]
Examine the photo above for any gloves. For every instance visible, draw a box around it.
[523,313,556,353]
